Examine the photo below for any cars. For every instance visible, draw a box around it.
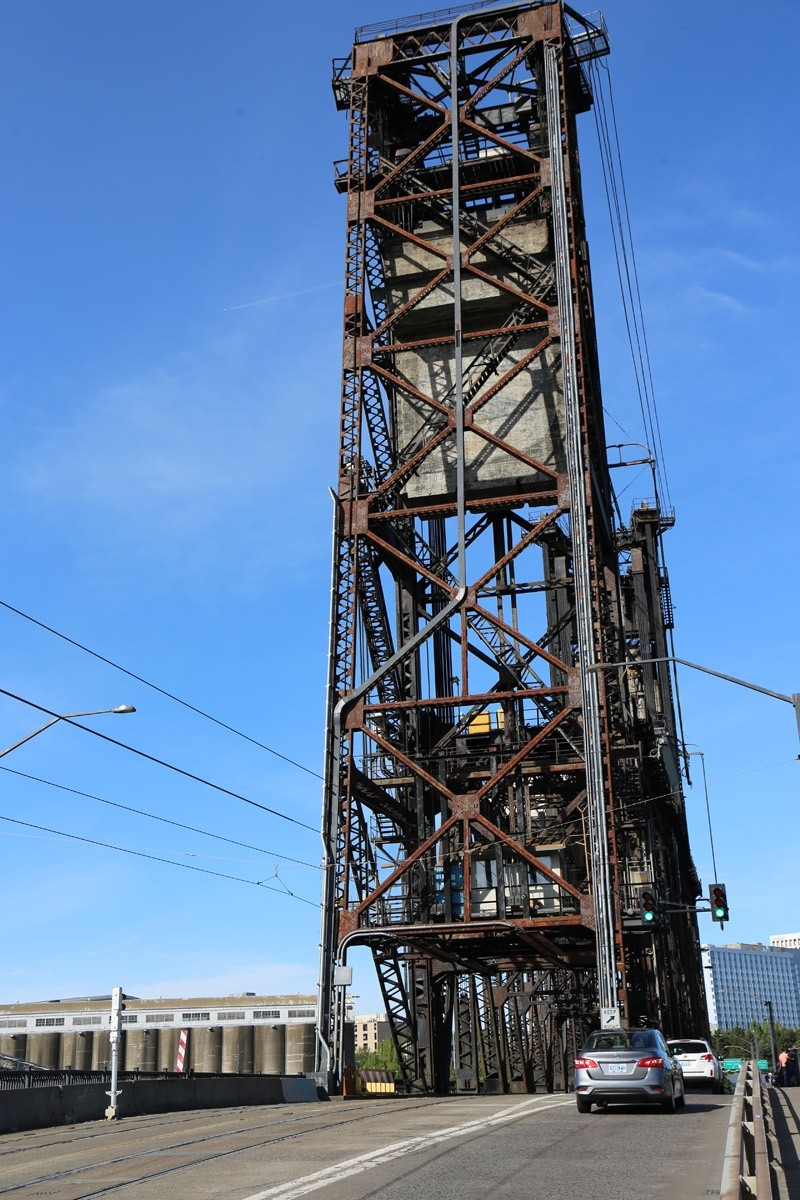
[666,1040,725,1095]
[574,1029,685,1114]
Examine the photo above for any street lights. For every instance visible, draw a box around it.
[717,989,778,1072]
[589,657,799,744]
[725,1004,759,1059]
[719,1033,754,1061]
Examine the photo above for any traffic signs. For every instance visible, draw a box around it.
[601,1007,621,1029]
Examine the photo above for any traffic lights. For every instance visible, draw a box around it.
[709,883,729,922]
[639,887,658,926]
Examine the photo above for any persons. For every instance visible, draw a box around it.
[778,1048,795,1086]
[765,1073,770,1088]
[789,1050,800,1087]
[771,1072,777,1088]
